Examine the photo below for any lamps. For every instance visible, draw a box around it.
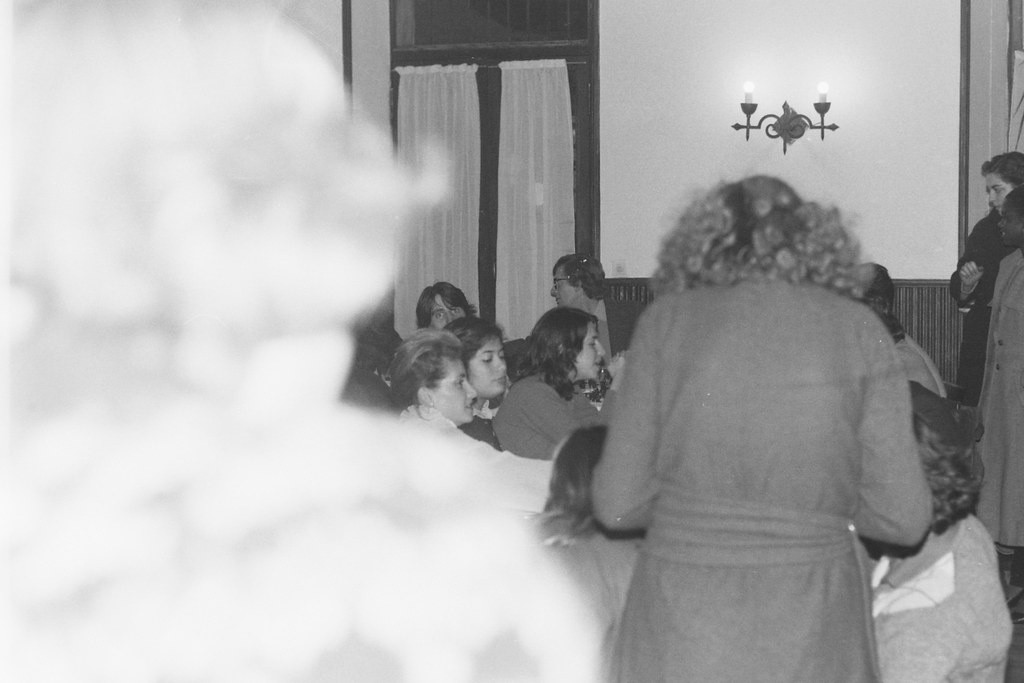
[731,80,839,155]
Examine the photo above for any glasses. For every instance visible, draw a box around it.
[553,278,568,290]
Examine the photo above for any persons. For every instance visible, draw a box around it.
[339,151,1023,683]
[587,175,934,683]
